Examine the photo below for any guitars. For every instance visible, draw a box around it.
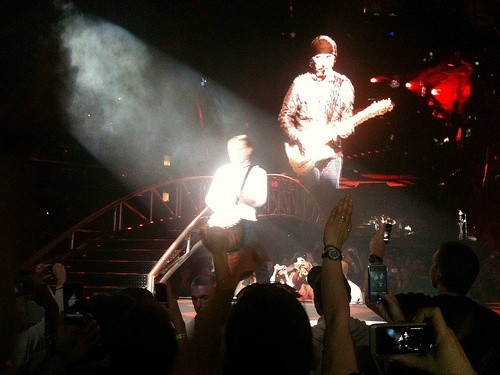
[281,99,395,174]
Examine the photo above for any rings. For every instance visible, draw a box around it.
[340,215,345,221]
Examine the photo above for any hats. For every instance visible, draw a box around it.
[310,35,337,58]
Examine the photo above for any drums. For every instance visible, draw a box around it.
[203,215,247,270]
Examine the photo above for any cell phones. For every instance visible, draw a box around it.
[64,281,85,317]
[367,264,388,304]
[369,322,437,356]
[383,224,393,244]
[155,284,169,303]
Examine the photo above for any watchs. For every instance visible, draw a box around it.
[368,254,383,265]
[321,248,342,261]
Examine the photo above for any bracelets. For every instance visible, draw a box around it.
[54,287,63,291]
[324,245,340,252]
[175,333,187,339]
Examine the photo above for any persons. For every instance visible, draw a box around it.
[279,36,354,196]
[203,134,266,271]
[0,197,500,375]
